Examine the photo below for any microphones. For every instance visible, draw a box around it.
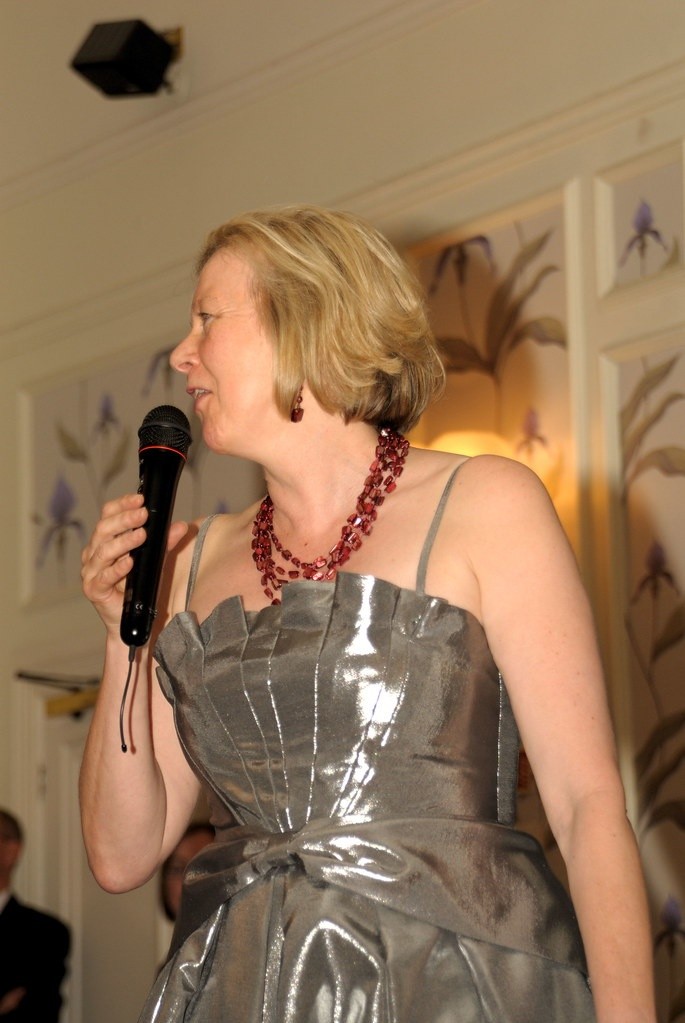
[118,406,192,755]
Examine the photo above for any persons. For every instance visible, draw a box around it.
[75,204,660,1023]
[152,818,217,986]
[0,805,74,1023]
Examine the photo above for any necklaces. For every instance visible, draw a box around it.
[250,426,411,606]
